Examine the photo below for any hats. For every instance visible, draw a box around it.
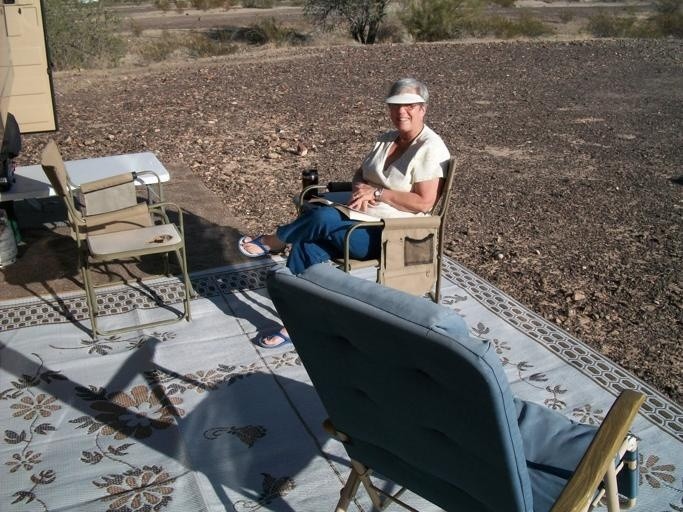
[384,86,426,105]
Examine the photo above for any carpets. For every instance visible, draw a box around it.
[0,254,683,512]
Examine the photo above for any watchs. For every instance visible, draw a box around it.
[374,187,383,201]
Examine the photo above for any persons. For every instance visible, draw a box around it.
[238,78,450,348]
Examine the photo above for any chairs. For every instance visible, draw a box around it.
[265,265,647,512]
[301,156,458,303]
[41,140,196,342]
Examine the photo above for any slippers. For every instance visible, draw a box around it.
[238,233,286,258]
[258,328,291,349]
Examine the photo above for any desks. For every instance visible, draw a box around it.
[0,152,170,203]
